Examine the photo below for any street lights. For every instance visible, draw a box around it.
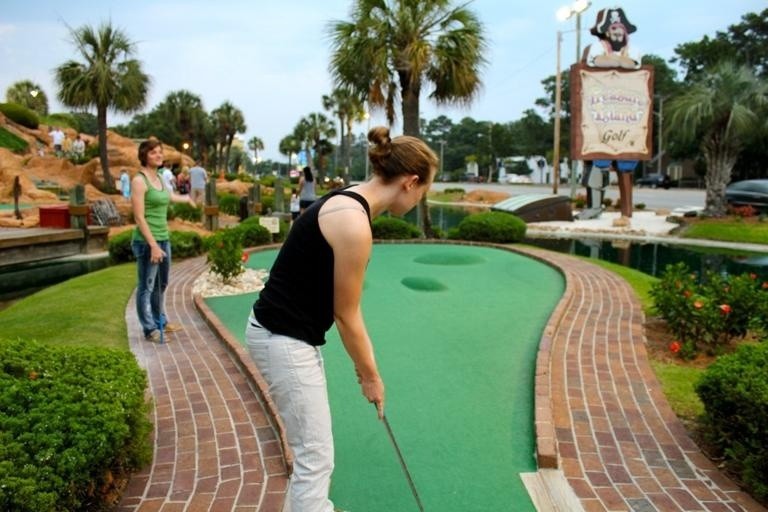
[556,0,591,200]
[364,113,370,182]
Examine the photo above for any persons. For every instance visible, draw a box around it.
[47,125,99,160]
[237,194,273,222]
[158,158,209,204]
[558,156,584,185]
[130,140,185,344]
[296,168,317,216]
[289,190,300,222]
[244,125,441,512]
[114,170,131,199]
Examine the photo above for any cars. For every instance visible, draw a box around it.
[635,173,671,189]
[726,178,768,214]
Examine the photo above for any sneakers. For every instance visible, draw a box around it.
[147,330,169,342]
[163,322,182,332]
